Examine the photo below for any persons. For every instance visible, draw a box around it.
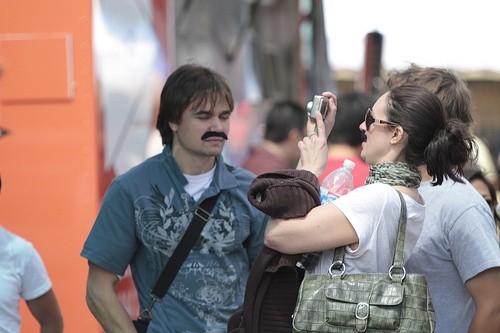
[0,125,63,333]
[387,62,500,333]
[241,91,500,240]
[81,58,293,333]
[263,85,480,333]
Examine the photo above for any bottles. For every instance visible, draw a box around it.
[292,159,355,270]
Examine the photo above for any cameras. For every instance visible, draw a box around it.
[307,95,329,120]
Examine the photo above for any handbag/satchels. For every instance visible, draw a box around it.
[292,190,436,333]
[133,318,151,333]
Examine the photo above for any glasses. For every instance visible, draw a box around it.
[366,108,406,132]
